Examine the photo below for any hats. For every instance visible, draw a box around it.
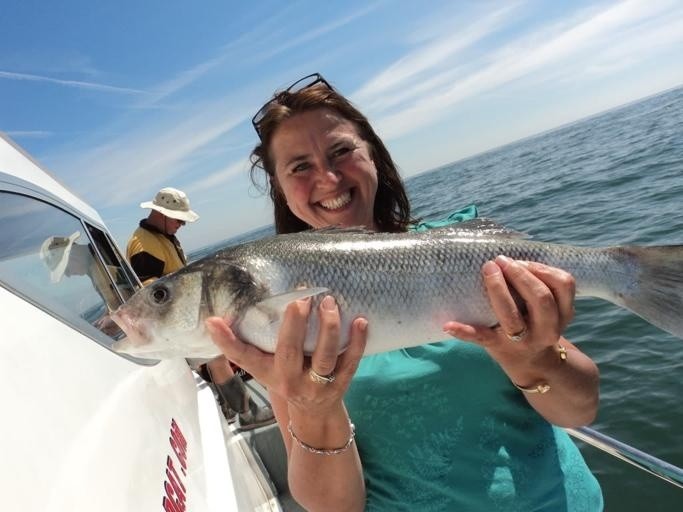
[139,186,200,224]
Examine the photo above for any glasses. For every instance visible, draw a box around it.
[251,72,336,145]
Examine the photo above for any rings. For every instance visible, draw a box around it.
[308,368,336,384]
[506,329,530,342]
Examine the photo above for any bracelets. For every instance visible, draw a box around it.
[508,342,567,393]
[288,418,355,456]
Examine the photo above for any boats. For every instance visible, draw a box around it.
[0,128,682,511]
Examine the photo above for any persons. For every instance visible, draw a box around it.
[122,188,277,432]
[38,230,122,337]
[200,76,602,512]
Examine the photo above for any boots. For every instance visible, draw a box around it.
[216,371,278,430]
